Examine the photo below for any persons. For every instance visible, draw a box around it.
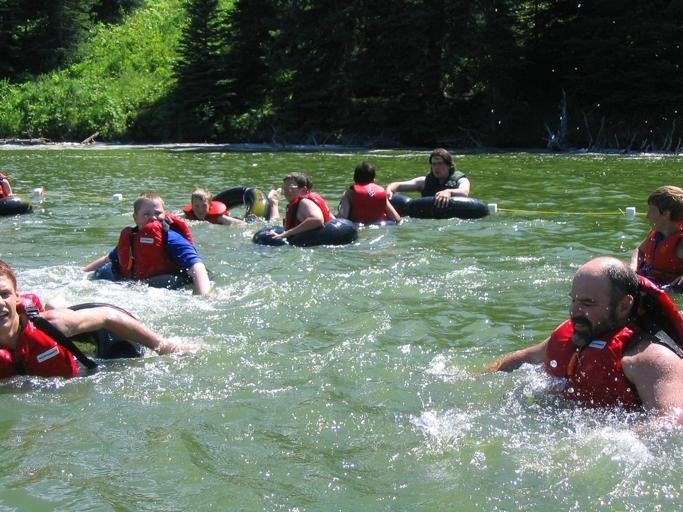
[175,188,282,228]
[0,260,194,389]
[0,172,12,201]
[630,186,682,291]
[385,149,471,210]
[82,193,210,296]
[265,171,335,240]
[334,161,402,223]
[481,256,683,437]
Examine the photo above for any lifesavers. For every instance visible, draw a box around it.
[91,259,211,290]
[339,191,412,228]
[67,302,144,357]
[252,216,358,247]
[407,194,488,219]
[0,195,33,218]
[212,186,270,224]
